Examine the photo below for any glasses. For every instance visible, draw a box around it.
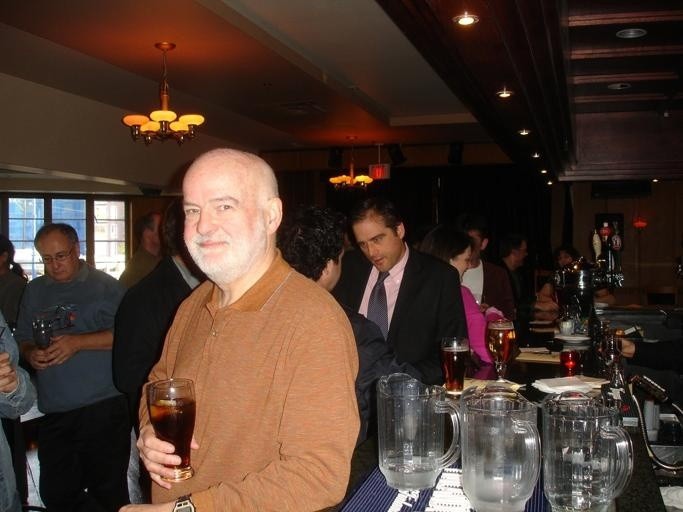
[41,245,76,264]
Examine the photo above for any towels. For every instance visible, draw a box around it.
[531,377,593,395]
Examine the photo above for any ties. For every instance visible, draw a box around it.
[366,272,390,343]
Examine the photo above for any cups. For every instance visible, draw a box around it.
[443,336,469,393]
[541,392,632,512]
[146,378,196,482]
[558,319,574,336]
[561,349,579,379]
[485,319,513,381]
[32,318,53,354]
[377,371,464,487]
[458,382,540,512]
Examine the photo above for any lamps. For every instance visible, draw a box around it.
[452,11,481,26]
[497,86,513,98]
[633,216,648,233]
[518,128,531,135]
[122,42,205,145]
[369,141,390,179]
[329,134,373,190]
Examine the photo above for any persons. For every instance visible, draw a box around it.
[495,232,561,311]
[334,198,469,385]
[16,222,130,512]
[417,221,494,363]
[111,199,205,492]
[117,149,360,512]
[456,215,516,323]
[0,312,37,512]
[277,205,425,450]
[614,338,683,372]
[537,244,616,305]
[120,211,162,288]
[0,235,28,331]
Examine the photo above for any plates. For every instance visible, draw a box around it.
[556,334,593,345]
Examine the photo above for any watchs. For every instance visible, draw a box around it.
[171,494,196,512]
